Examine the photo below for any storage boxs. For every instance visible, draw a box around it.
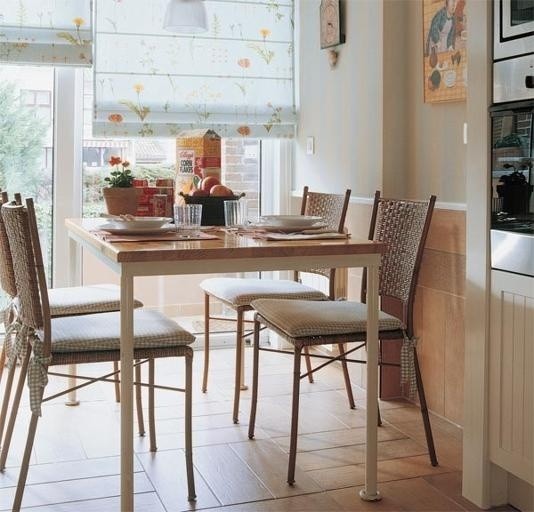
[176,129,222,183]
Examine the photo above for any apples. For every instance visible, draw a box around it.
[210,184,234,196]
[201,176,220,190]
[189,189,210,197]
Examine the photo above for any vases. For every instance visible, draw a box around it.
[103,187,139,215]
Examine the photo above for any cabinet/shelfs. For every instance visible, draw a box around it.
[461,0,533,512]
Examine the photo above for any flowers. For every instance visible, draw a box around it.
[104,156,135,187]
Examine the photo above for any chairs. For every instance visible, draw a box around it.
[249,190,438,486]
[199,185,356,425]
[1,190,147,439]
[1,193,196,512]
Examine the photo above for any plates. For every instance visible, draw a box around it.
[258,215,323,226]
[256,222,329,234]
[105,216,174,229]
[95,224,176,236]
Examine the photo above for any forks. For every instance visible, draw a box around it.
[276,229,305,235]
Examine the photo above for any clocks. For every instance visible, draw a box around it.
[320,0,346,68]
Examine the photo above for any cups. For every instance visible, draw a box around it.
[173,205,191,236]
[223,199,246,227]
[153,194,167,217]
[183,203,203,239]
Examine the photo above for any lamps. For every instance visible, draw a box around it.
[164,0,210,33]
